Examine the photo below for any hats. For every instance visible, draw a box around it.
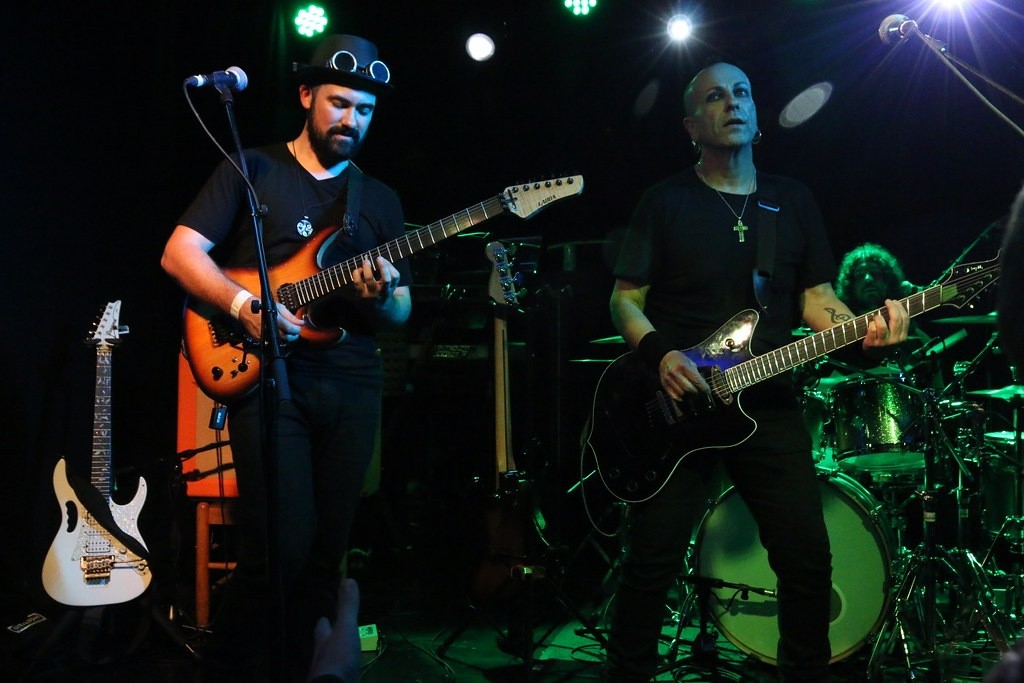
[295,34,395,95]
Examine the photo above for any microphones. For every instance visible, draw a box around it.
[879,14,918,45]
[186,66,248,91]
[911,337,942,359]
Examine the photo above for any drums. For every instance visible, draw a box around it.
[981,431,1024,540]
[831,372,941,469]
[839,460,957,494]
[801,378,839,472]
[690,464,899,669]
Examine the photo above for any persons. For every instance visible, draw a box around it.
[836,244,944,400]
[162,33,412,662]
[308,578,362,683]
[606,63,911,683]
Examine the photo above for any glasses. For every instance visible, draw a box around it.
[330,51,390,83]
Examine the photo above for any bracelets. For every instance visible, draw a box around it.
[230,290,254,320]
[638,331,680,370]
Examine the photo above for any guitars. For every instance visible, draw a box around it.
[587,247,1010,503]
[466,239,534,616]
[40,300,154,606]
[182,170,583,402]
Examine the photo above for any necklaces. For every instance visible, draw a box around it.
[293,141,342,237]
[699,165,755,243]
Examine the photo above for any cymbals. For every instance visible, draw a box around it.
[569,359,612,363]
[930,308,1001,324]
[791,326,816,336]
[589,334,624,344]
[962,383,1024,402]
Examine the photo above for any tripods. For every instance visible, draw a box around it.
[436,500,780,683]
[816,309,1024,683]
